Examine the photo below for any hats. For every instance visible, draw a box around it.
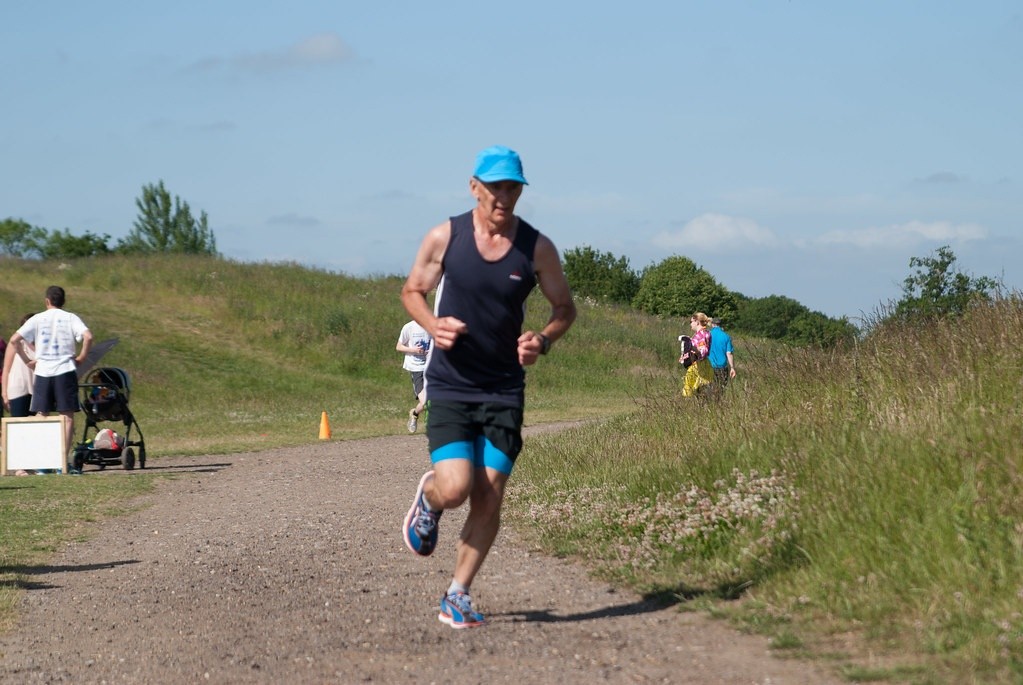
[473,147,529,186]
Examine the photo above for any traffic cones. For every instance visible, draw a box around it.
[318,411,331,439]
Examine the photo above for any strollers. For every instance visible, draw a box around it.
[68,359,147,472]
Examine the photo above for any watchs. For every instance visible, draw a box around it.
[538,333,551,355]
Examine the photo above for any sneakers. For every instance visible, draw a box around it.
[438,590,487,629]
[402,470,442,557]
[408,408,419,433]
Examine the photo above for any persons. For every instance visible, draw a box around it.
[89,375,110,403]
[396,321,431,433]
[10,285,93,475]
[0,312,37,475]
[403,146,576,627]
[706,319,736,393]
[681,313,715,397]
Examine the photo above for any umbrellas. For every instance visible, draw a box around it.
[77,336,121,391]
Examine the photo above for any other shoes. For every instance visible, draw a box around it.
[37,469,52,475]
[57,464,83,475]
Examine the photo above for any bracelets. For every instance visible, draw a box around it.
[731,367,734,368]
